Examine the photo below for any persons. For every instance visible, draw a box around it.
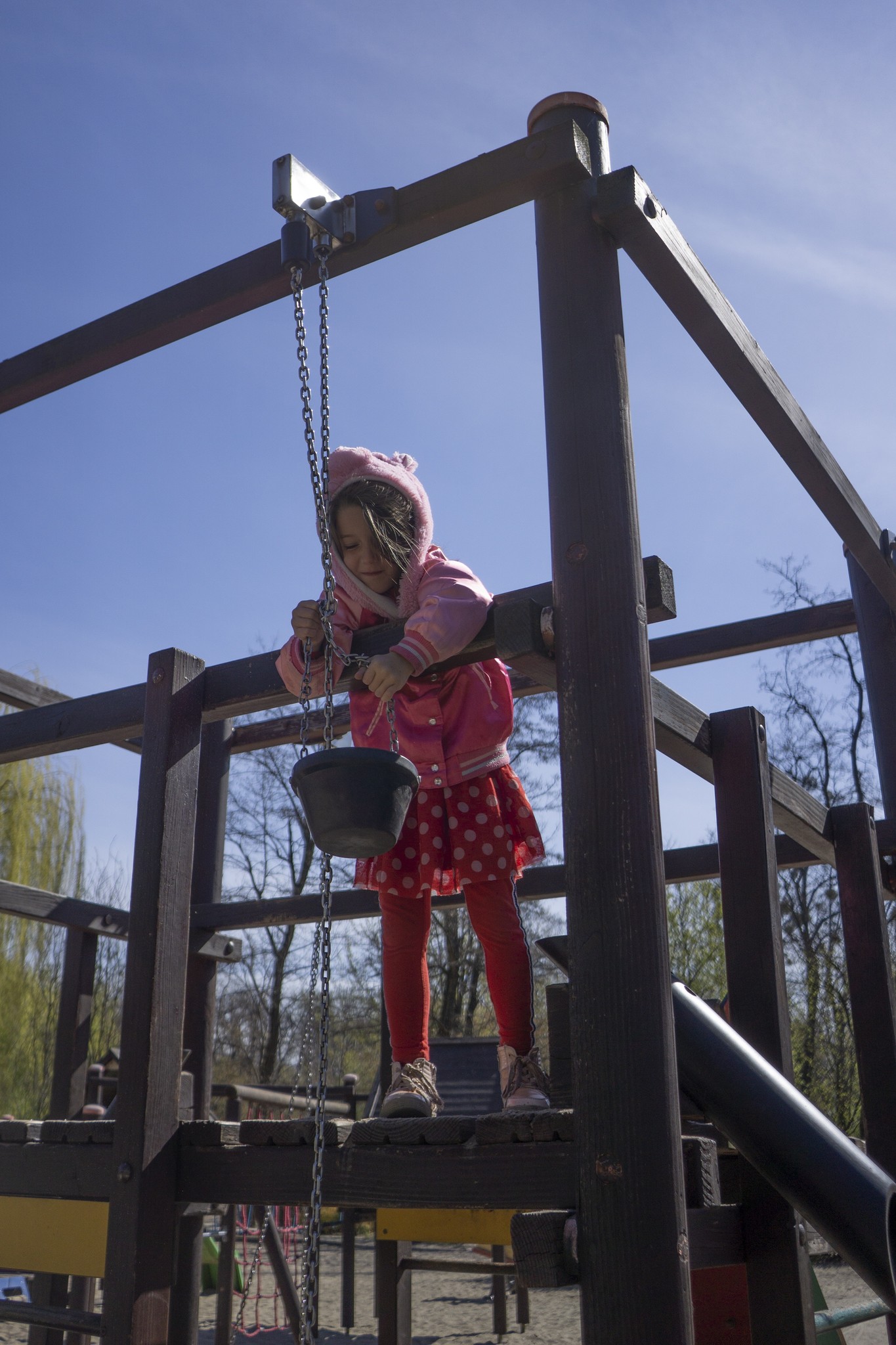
[274,448,547,1117]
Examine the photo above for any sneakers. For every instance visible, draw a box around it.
[495,1045,552,1112]
[380,1058,444,1116]
